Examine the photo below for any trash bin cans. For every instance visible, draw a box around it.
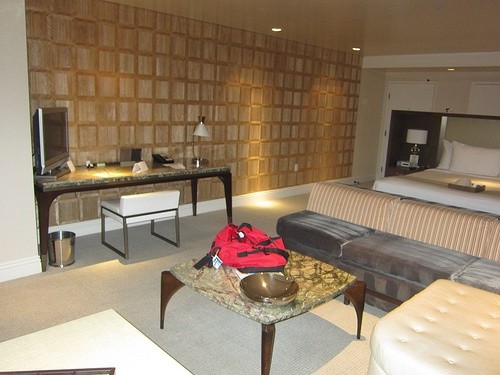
[47,231,76,267]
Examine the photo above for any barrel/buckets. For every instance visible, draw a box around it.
[47,231,76,266]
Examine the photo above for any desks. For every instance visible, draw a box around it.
[34,158,233,271]
[0,309,192,375]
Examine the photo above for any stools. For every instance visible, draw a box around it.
[370,278,500,375]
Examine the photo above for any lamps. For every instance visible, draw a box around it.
[401,129,430,166]
[192,116,211,165]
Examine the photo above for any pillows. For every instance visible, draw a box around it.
[449,140,500,177]
[436,139,454,169]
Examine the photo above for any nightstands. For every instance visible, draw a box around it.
[386,161,432,178]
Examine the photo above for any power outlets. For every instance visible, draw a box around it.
[295,164,300,171]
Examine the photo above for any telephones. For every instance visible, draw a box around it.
[152,153,174,164]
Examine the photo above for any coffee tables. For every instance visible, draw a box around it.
[160,244,366,375]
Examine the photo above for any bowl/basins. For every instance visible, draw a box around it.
[240,273,299,306]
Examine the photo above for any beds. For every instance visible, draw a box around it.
[372,167,500,218]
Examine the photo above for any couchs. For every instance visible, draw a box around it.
[276,181,500,315]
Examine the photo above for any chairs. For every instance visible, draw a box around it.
[100,190,183,260]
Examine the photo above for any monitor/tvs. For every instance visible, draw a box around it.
[32,106,71,179]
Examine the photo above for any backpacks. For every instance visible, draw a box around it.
[193,222,290,274]
